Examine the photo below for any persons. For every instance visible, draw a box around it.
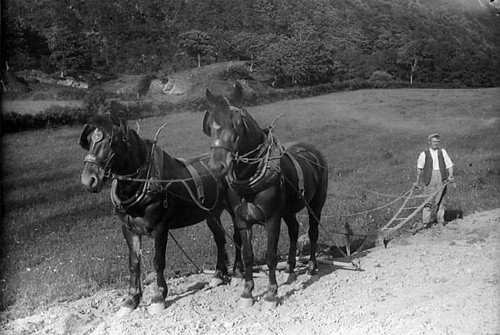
[416,133,455,229]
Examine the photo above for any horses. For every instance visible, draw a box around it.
[203,89,328,311]
[79,101,245,316]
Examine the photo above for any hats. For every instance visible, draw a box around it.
[428,133,439,141]
